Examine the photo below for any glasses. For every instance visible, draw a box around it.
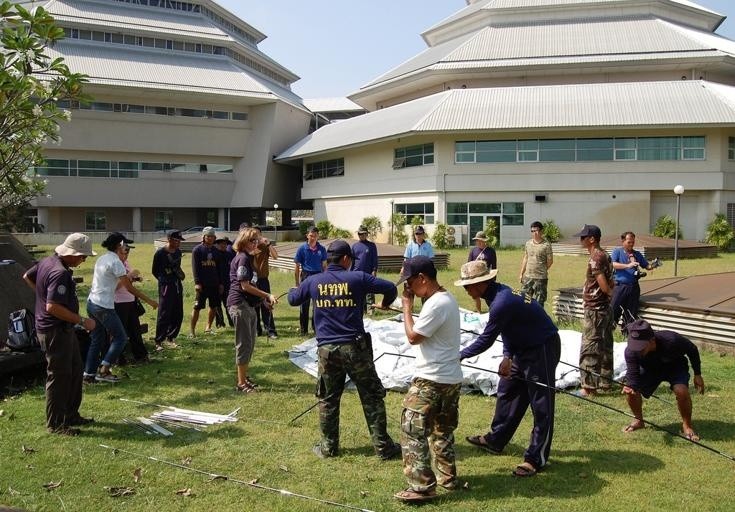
[248,239,259,244]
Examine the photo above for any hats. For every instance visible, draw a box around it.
[167,229,185,240]
[356,226,369,234]
[327,241,360,260]
[454,260,498,286]
[201,221,250,245]
[414,226,424,235]
[54,233,97,257]
[394,255,434,286]
[472,231,490,242]
[628,319,654,351]
[573,224,601,237]
[108,232,135,251]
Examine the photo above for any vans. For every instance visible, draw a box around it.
[182,225,223,232]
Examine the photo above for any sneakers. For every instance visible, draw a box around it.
[376,443,400,460]
[48,427,81,437]
[313,445,327,458]
[69,416,94,426]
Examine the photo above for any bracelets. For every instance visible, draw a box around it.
[79,317,85,325]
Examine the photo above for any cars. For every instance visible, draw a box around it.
[252,224,268,231]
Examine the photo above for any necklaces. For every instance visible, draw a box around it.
[424,285,443,302]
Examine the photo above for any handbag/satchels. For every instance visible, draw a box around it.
[135,295,145,317]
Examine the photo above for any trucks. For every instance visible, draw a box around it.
[289,220,314,230]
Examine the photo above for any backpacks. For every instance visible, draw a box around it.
[7,307,37,348]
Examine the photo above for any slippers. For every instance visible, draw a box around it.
[621,425,635,433]
[685,433,700,443]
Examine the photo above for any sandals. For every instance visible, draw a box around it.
[466,435,505,455]
[513,465,537,477]
[95,372,120,383]
[236,379,257,393]
[393,487,438,500]
[438,477,457,490]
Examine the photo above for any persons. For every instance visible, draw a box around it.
[22,232,97,436]
[610,230,657,338]
[573,226,616,397]
[620,321,704,440]
[393,254,463,501]
[83,233,158,382]
[287,239,400,461]
[295,227,327,333]
[458,259,561,479]
[153,226,236,351]
[401,225,434,313]
[468,231,497,312]
[518,221,553,308]
[350,226,377,316]
[226,226,277,393]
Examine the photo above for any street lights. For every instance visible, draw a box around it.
[674,185,684,276]
[274,203,278,240]
[389,198,394,245]
